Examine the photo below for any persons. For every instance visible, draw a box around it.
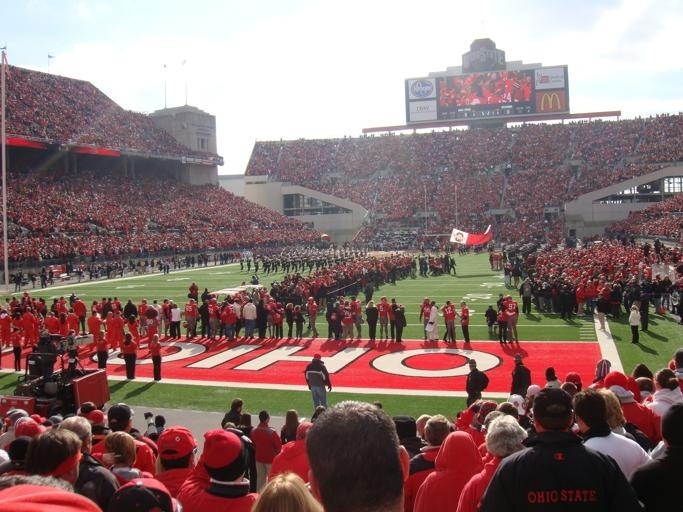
[8,348,683,512]
[303,353,332,409]
[0,65,683,382]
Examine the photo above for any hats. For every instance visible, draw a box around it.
[533,387,574,420]
[13,417,45,439]
[565,372,582,386]
[154,425,199,460]
[109,477,183,512]
[30,414,47,424]
[604,371,628,390]
[107,403,135,429]
[87,409,105,427]
[390,414,417,439]
[507,394,527,415]
[526,385,542,400]
[294,421,313,437]
[202,429,251,481]
[8,409,29,425]
[477,401,498,424]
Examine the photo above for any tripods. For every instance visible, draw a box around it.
[61,352,86,388]
